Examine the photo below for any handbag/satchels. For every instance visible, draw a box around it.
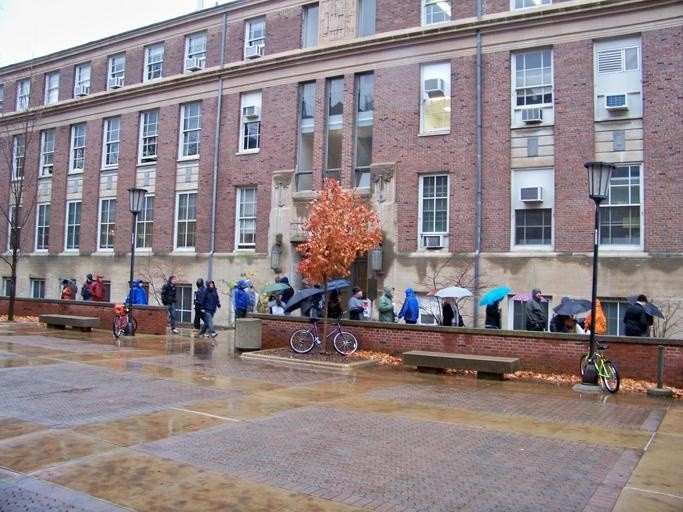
[625,319,641,333]
[550,315,564,332]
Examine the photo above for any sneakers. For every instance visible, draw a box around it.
[172,329,218,338]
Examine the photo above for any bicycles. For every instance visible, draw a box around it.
[288,312,358,356]
[113,302,138,338]
[579,342,620,394]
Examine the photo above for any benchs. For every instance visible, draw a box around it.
[34,313,100,334]
[400,350,521,380]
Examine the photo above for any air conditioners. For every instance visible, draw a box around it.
[422,75,634,128]
[70,43,263,98]
[241,105,258,119]
[518,183,544,205]
[421,234,443,250]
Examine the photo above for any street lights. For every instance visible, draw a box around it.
[580,161,613,384]
[122,186,146,336]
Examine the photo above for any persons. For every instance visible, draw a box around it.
[161,275,179,333]
[623,295,653,337]
[485,300,501,329]
[269,292,290,315]
[301,293,326,317]
[327,289,342,319]
[348,287,364,320]
[233,280,255,318]
[61,279,77,300]
[441,297,464,327]
[526,288,606,335]
[378,284,397,322]
[396,287,419,324]
[89,276,106,302]
[192,278,221,337]
[81,274,92,300]
[126,280,148,304]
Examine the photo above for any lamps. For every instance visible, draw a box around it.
[268,241,284,273]
[370,245,384,277]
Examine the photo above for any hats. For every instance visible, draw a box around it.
[354,288,364,292]
[637,295,647,301]
